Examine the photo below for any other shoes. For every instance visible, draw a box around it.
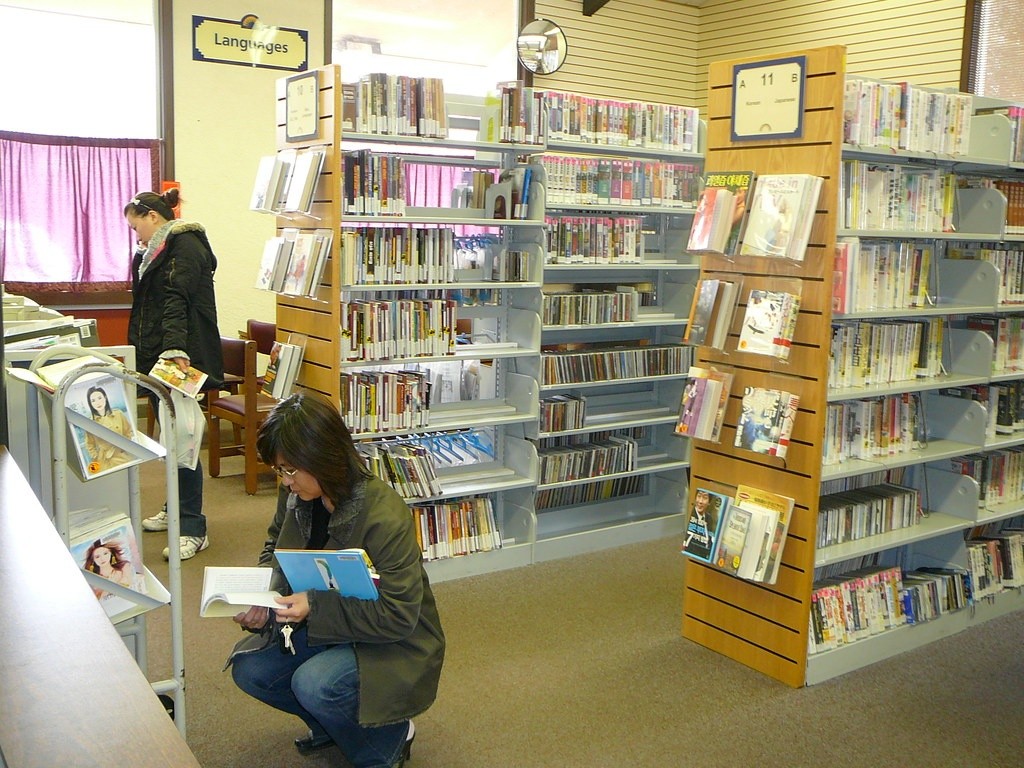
[389,731,415,768]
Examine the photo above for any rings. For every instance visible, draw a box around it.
[250,620,257,624]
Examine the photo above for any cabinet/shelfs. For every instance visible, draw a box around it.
[680,45,1024,691]
[275,62,707,583]
[0,293,137,662]
[25,343,188,742]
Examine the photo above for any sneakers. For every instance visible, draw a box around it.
[142,510,168,531]
[162,532,210,560]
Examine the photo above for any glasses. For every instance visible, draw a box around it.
[270,466,298,478]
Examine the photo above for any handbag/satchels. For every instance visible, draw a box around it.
[159,388,206,471]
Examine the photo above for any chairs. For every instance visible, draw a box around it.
[237,319,276,430]
[146,374,243,445]
[205,335,274,496]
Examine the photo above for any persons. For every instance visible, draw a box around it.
[220,388,445,768]
[123,188,225,559]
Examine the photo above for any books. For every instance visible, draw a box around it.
[354,440,443,498]
[200,566,288,618]
[51,507,149,618]
[407,499,504,562]
[261,341,301,399]
[674,79,1024,658]
[340,299,458,363]
[274,548,381,601]
[2,289,144,479]
[148,357,209,400]
[249,152,322,213]
[341,150,407,217]
[340,369,432,435]
[342,226,454,286]
[454,80,700,511]
[254,234,330,297]
[342,73,449,139]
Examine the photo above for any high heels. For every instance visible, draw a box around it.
[295,726,335,752]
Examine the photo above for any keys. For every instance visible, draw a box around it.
[281,616,296,656]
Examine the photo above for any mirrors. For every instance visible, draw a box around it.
[516,17,567,75]
